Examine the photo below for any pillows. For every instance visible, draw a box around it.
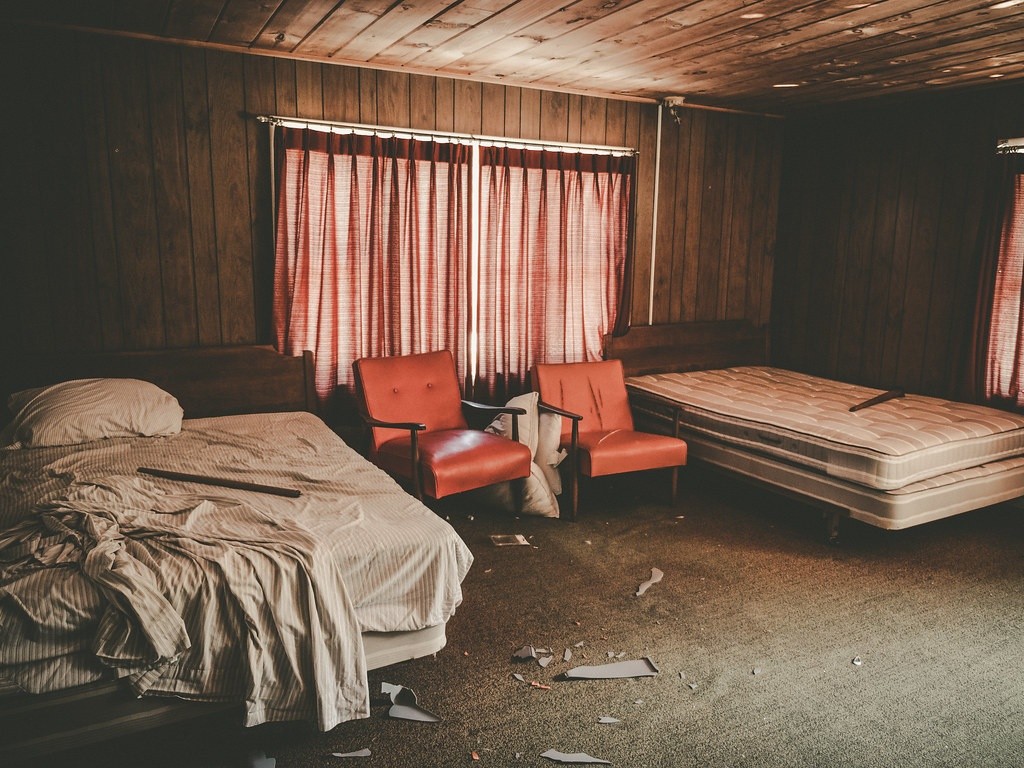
[534,411,568,494]
[3,378,184,450]
[482,461,560,518]
[483,391,539,461]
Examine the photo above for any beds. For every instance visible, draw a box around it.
[604,318,1024,543]
[0,346,474,765]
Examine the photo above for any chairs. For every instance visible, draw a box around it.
[352,349,532,521]
[528,359,687,523]
